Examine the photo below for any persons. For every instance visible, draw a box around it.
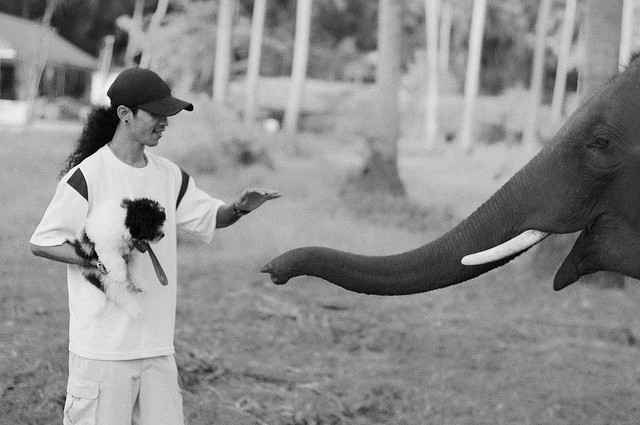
[29,67,284,424]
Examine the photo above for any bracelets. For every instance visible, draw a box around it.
[96,257,108,275]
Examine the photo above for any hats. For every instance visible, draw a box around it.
[107,67,193,116]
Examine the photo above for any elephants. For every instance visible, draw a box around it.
[259,45,640,296]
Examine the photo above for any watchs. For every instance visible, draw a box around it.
[231,201,251,217]
